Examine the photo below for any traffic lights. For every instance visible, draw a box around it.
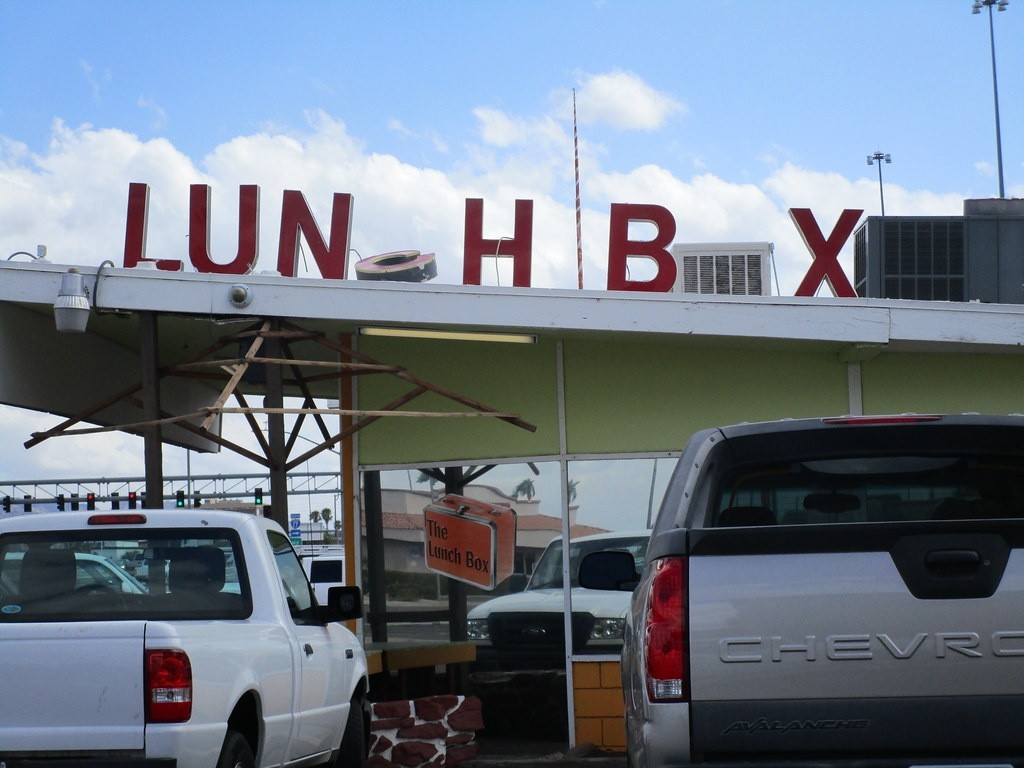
[129,491,136,509]
[2,495,10,513]
[87,492,95,510]
[254,488,263,505]
[194,491,201,508]
[177,491,184,507]
[55,493,65,511]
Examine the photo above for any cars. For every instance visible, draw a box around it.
[464,529,654,654]
[4,550,149,596]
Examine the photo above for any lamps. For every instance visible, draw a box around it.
[53,267,90,333]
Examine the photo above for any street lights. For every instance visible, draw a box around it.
[971,1,1012,199]
[867,150,891,215]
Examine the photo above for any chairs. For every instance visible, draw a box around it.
[578,551,637,593]
[15,548,82,614]
[717,505,778,529]
[970,489,1024,521]
[158,546,244,611]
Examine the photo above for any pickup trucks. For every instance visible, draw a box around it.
[134,558,169,585]
[622,400,1024,768]
[223,557,346,605]
[0,514,372,768]
